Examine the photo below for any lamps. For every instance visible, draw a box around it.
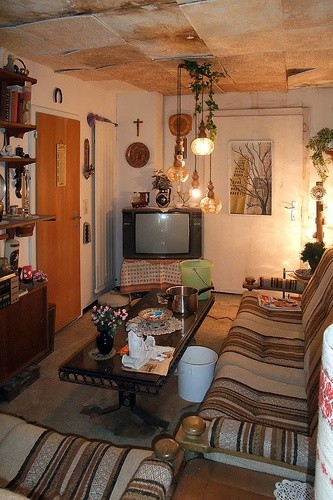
[166,62,222,215]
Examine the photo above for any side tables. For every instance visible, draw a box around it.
[171,458,315,500]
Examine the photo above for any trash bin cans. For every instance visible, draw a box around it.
[177,346,218,403]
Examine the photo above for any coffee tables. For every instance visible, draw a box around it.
[59,289,212,428]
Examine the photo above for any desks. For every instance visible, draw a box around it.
[289,268,315,283]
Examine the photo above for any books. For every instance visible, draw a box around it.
[257,294,301,311]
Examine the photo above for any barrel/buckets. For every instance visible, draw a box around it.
[179,258,214,300]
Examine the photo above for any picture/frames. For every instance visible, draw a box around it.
[228,140,273,216]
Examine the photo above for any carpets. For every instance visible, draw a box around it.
[0,324,223,453]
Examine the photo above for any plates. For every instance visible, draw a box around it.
[138,308,173,322]
[125,142,150,168]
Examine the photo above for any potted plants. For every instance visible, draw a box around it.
[305,127,333,182]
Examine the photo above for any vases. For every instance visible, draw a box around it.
[308,259,318,271]
[156,188,171,208]
[94,326,113,355]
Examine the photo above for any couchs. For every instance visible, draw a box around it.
[175,247,333,468]
[0,415,179,500]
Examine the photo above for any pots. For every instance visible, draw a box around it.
[160,285,214,316]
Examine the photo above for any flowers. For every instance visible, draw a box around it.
[152,168,173,189]
[301,241,327,264]
[91,303,128,335]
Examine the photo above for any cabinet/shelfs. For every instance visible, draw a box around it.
[0,67,50,388]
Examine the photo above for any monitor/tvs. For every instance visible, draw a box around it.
[122,208,203,259]
